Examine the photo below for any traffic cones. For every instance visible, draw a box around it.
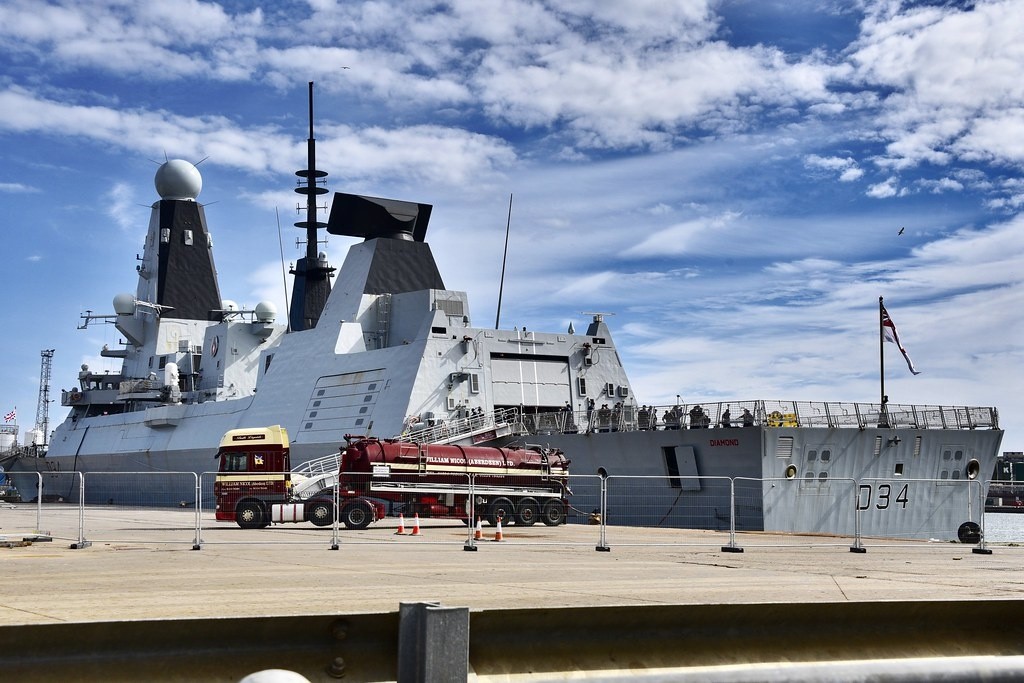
[473,516,485,541]
[393,513,408,535]
[490,517,506,542]
[408,512,423,536]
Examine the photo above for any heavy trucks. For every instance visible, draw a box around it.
[214,424,573,528]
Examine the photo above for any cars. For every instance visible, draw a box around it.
[1011,500,1023,506]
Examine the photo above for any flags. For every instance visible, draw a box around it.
[4,409,16,423]
[882,305,920,374]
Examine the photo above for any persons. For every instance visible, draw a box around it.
[31,441,38,456]
[561,403,754,436]
[468,406,484,430]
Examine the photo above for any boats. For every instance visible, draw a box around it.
[0,80,1004,545]
[1,489,20,502]
